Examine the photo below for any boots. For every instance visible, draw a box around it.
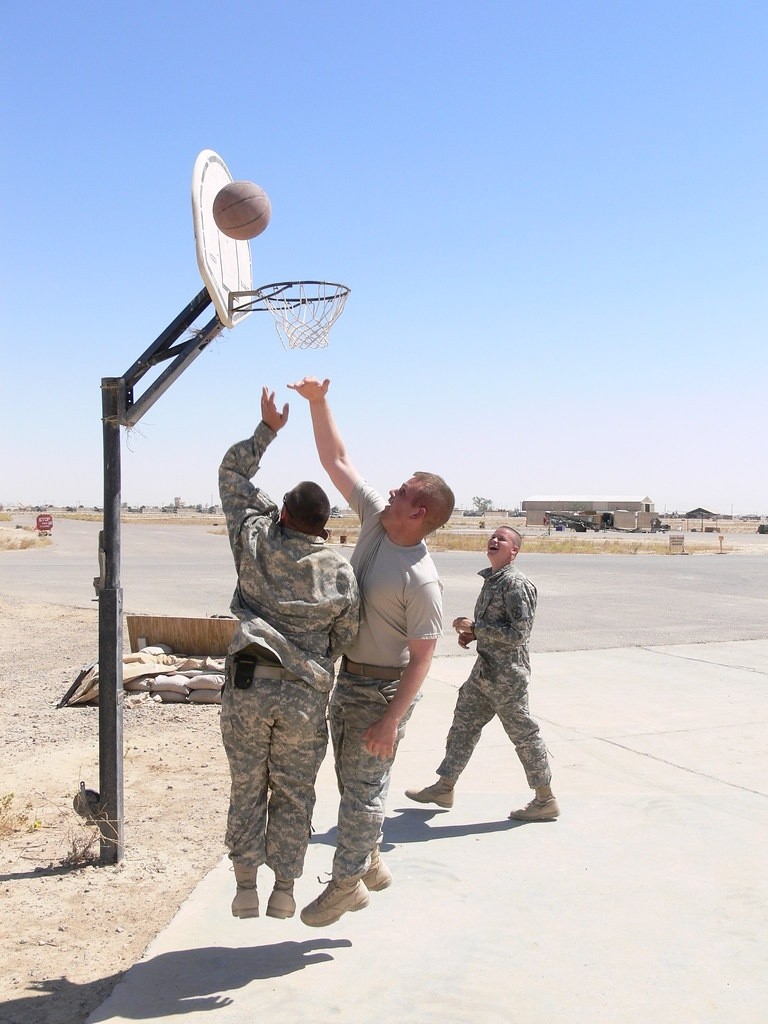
[509,784,560,822]
[300,846,392,927]
[404,776,455,808]
[266,882,296,919]
[231,863,259,920]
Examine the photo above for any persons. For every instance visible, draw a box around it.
[219,387,362,919]
[406,526,561,821]
[287,376,454,925]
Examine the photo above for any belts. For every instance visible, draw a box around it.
[345,661,406,681]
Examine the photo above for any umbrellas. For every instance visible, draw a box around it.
[686,508,715,531]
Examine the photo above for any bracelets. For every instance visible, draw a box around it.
[471,622,475,632]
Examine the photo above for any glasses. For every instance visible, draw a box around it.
[283,492,294,518]
